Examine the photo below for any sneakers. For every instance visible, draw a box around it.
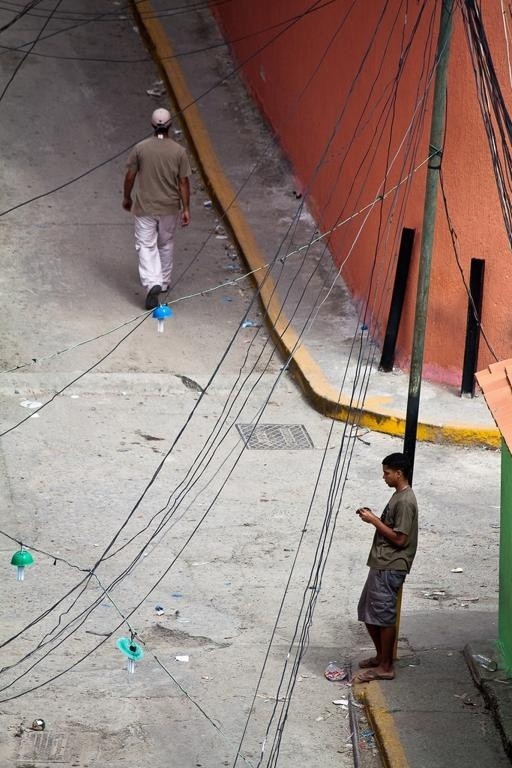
[146,284,170,310]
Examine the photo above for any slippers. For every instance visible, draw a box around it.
[357,670,395,681]
[359,657,380,667]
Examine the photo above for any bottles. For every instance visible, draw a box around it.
[472,654,497,672]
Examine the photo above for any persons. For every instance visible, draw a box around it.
[120,107,194,310]
[357,452,418,684]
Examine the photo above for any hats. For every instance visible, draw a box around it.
[151,108,172,128]
